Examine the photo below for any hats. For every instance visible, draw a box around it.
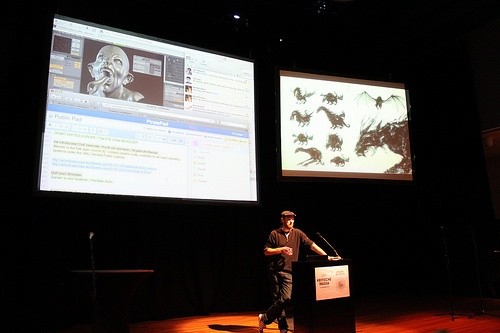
[281,211,296,217]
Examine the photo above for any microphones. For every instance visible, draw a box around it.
[316,232,342,261]
[88,231,94,239]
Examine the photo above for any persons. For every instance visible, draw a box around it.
[258,210,341,333]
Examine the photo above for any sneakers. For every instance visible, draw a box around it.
[258,313,265,333]
[280,329,288,333]
